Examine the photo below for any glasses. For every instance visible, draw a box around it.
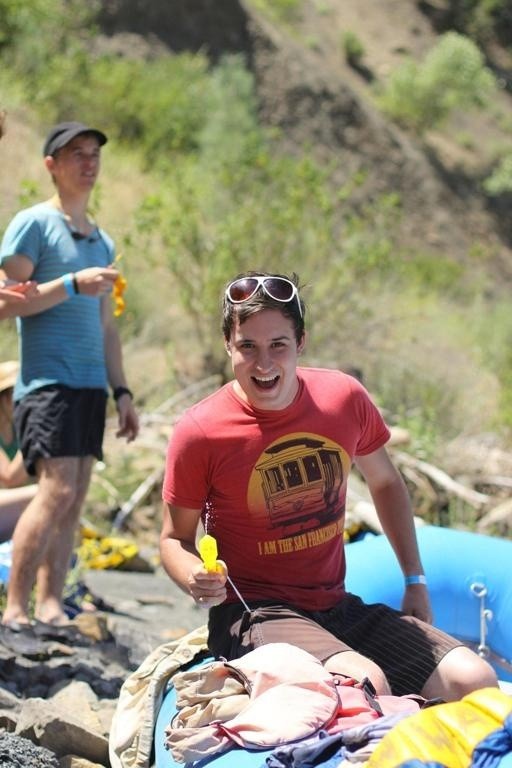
[225,277,302,318]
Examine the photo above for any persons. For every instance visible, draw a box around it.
[0,120,139,631]
[160,272,499,702]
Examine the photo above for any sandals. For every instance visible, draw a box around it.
[62,581,114,619]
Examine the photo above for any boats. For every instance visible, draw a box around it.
[142,517,512,768]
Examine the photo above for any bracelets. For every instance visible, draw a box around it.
[404,575,426,586]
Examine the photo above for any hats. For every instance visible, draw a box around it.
[44,122,107,156]
[0,360,20,392]
